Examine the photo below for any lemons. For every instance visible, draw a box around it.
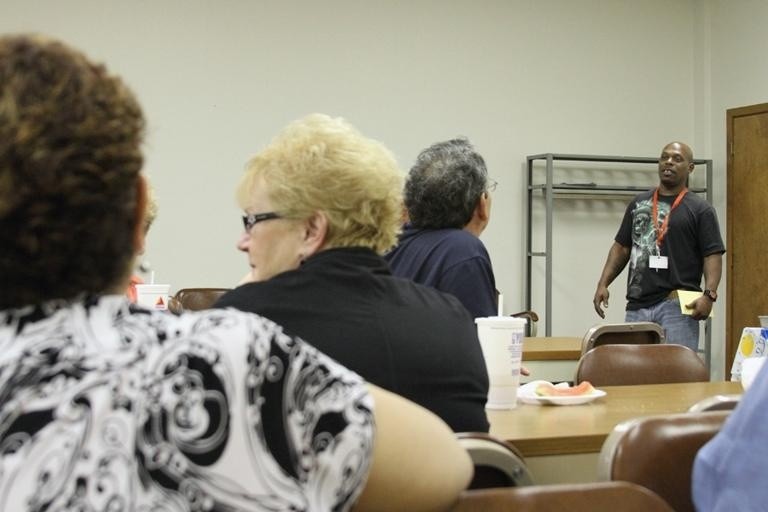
[740,333,755,357]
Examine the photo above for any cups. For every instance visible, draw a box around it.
[739,357,767,391]
[134,283,171,314]
[472,315,527,410]
[757,315,768,327]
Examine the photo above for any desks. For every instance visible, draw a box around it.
[520,336,585,381]
[482,381,743,488]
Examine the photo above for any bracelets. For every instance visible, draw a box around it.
[703,289,718,302]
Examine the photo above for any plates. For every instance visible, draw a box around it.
[522,389,607,408]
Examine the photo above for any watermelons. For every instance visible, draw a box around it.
[534,380,596,399]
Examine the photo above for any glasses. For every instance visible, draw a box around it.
[483,177,497,194]
[242,211,276,230]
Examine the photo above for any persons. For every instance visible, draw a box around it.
[211,110,492,438]
[1,33,474,511]
[692,349,768,511]
[591,141,723,353]
[384,134,500,321]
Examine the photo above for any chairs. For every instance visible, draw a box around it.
[453,433,532,489]
[495,288,538,335]
[575,344,706,386]
[600,410,729,512]
[688,395,741,412]
[173,288,232,310]
[458,484,672,512]
[581,322,664,359]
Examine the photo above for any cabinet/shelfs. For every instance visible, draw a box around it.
[524,152,714,383]
